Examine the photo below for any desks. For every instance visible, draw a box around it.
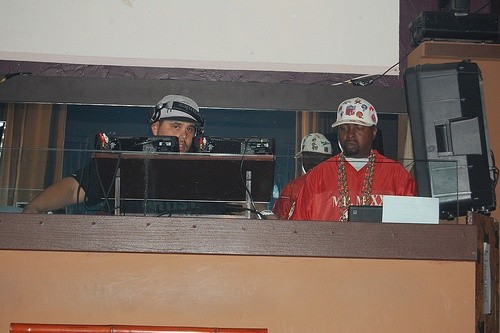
[0,211,484,333]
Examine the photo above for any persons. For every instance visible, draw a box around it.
[269,133,332,220]
[285,97,419,222]
[22,94,200,213]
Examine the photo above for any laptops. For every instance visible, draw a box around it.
[347,205,383,222]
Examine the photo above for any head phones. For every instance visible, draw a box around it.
[148,101,206,136]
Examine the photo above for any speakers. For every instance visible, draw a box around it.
[403,62,496,221]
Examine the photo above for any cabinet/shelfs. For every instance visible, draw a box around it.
[407,42,500,333]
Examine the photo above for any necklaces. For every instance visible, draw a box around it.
[337,148,375,222]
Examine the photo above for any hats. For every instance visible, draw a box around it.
[149,95,204,135]
[331,97,378,128]
[293,133,332,158]
